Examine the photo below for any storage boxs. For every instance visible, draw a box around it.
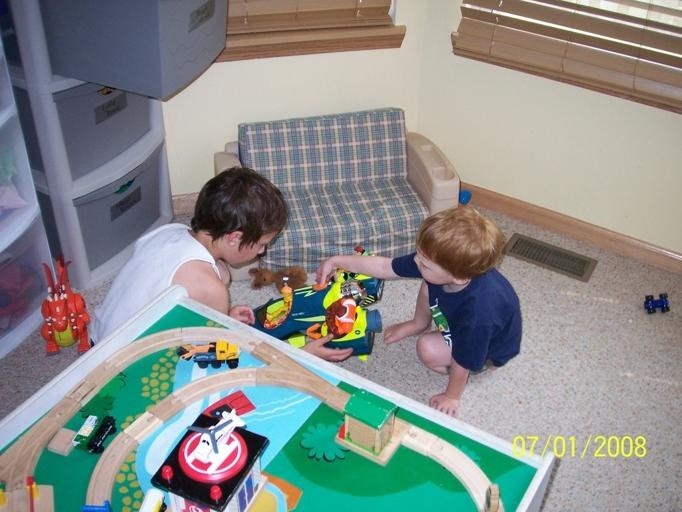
[36,144,162,273]
[0,116,36,236]
[12,65,152,184]
[0,51,18,111]
[0,219,55,339]
[0,0,229,103]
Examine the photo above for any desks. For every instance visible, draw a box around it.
[0,284,559,511]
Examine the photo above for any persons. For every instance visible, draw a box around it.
[315,205,522,420]
[89,165,353,362]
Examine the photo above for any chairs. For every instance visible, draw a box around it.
[213,105,463,278]
